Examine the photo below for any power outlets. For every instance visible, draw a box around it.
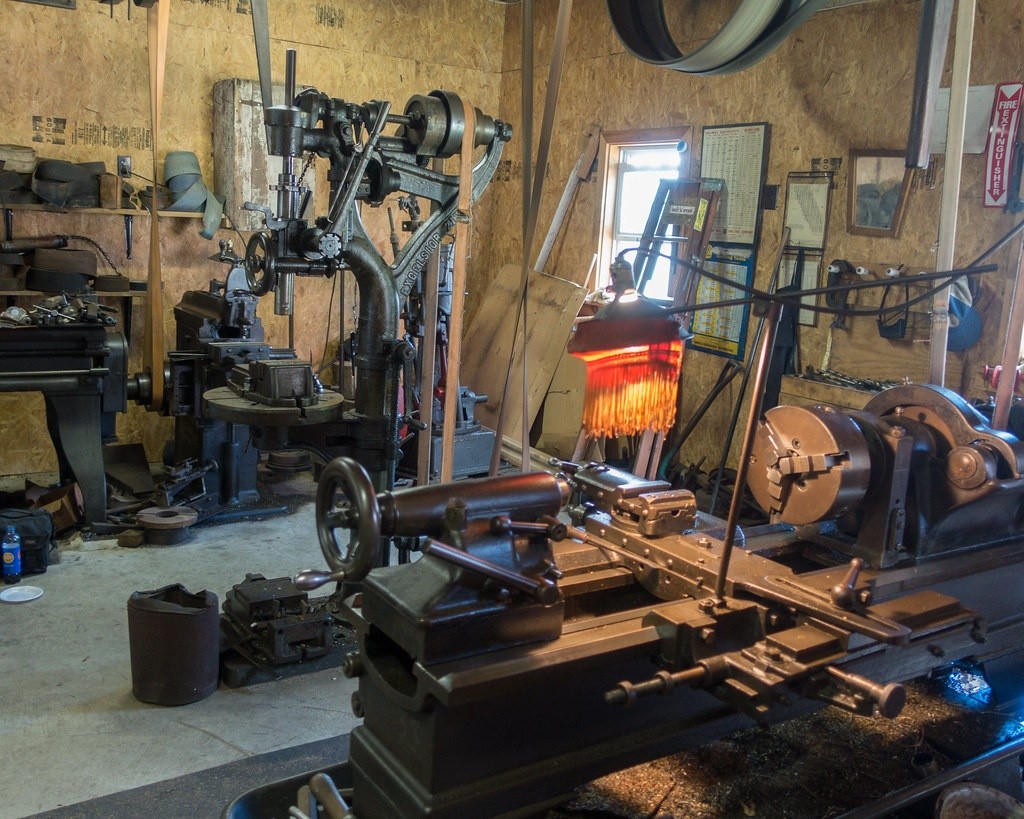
[117,156,133,178]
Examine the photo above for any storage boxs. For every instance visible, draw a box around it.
[31,484,84,532]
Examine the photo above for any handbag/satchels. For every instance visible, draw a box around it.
[0,508,58,574]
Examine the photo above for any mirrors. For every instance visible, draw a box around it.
[848,149,915,238]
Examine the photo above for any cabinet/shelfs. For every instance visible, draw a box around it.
[0,202,224,343]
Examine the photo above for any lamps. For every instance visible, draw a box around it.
[565,248,999,604]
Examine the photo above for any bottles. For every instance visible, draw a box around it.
[1,524,22,585]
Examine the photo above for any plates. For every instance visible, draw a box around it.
[0,586,44,603]
